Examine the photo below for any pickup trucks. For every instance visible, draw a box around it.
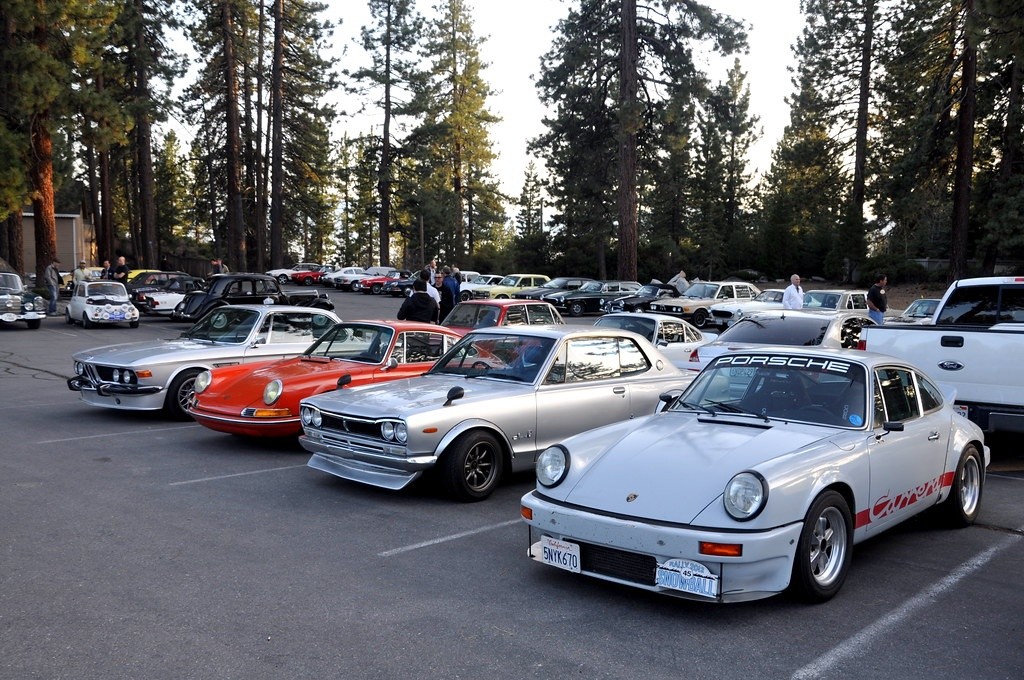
[857,276,1024,438]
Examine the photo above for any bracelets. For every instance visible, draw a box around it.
[877,309,879,311]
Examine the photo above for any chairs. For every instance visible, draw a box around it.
[753,372,865,416]
[825,297,836,309]
[520,346,547,379]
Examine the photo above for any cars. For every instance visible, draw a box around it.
[520,347,990,606]
[429,299,568,361]
[381,269,485,298]
[67,304,403,420]
[320,267,413,295]
[171,272,336,331]
[599,285,661,313]
[460,275,515,302]
[511,275,605,311]
[885,298,941,324]
[292,264,339,286]
[543,282,649,316]
[58,269,208,323]
[705,288,822,330]
[645,280,775,328]
[594,313,718,365]
[186,319,507,438]
[298,326,732,502]
[265,262,319,284]
[66,280,140,328]
[472,274,551,299]
[0,271,46,330]
[801,290,889,315]
[687,309,878,394]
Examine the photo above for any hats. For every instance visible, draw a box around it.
[80,260,86,264]
[443,267,450,273]
[53,257,61,263]
[453,268,460,274]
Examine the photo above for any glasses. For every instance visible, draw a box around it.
[434,277,442,279]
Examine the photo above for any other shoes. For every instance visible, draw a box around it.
[49,311,57,316]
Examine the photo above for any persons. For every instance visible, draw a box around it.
[782,275,804,309]
[866,274,888,325]
[46,258,60,316]
[397,258,460,326]
[159,255,173,271]
[113,257,128,288]
[217,258,229,274]
[207,259,220,275]
[73,260,92,286]
[100,260,116,279]
